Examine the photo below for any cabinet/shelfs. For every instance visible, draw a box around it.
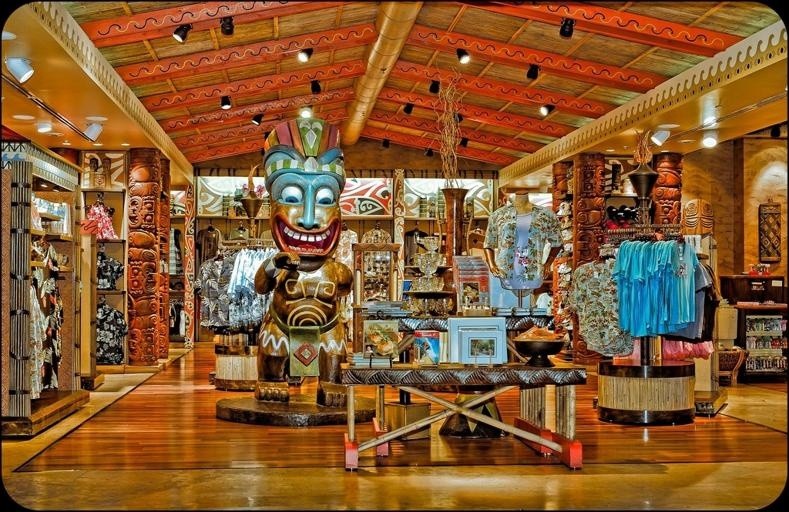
[542,142,789,439]
[0,140,193,439]
[196,166,540,428]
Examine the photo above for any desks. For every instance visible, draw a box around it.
[341,368,588,472]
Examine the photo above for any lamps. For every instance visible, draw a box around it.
[5,56,34,84]
[171,16,322,126]
[382,18,574,156]
[651,130,719,148]
[83,123,104,141]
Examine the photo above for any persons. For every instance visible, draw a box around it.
[480,190,565,291]
[249,117,356,407]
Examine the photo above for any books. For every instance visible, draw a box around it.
[449,255,492,315]
